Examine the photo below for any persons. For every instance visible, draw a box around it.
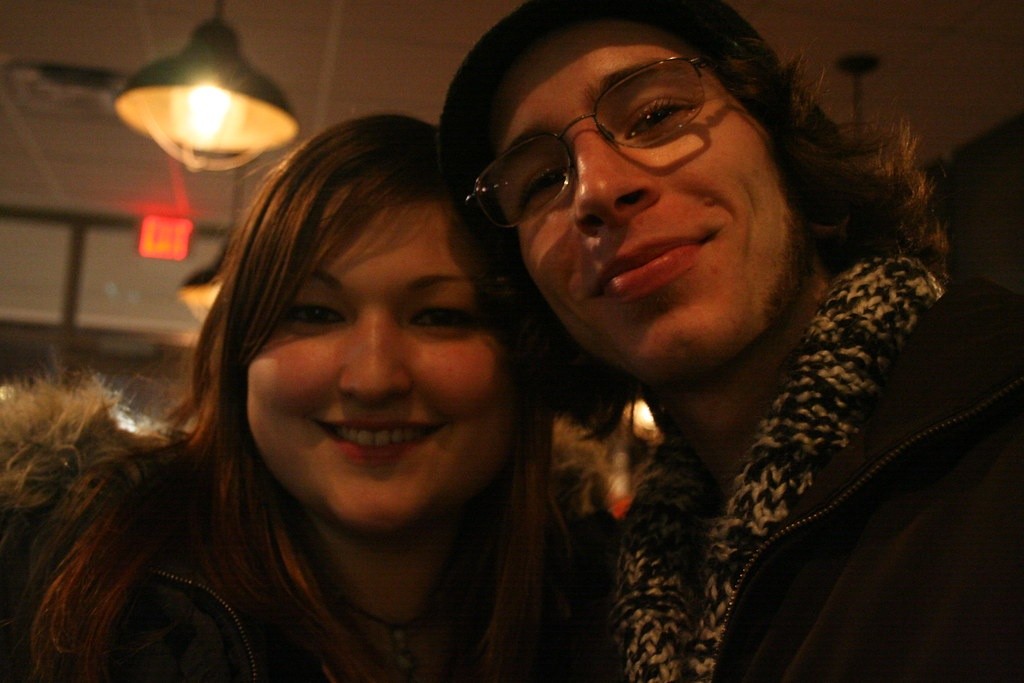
[439,0,1024,683]
[0,113,620,683]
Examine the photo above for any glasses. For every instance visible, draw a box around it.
[465,55,713,229]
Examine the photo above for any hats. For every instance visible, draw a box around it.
[434,0,779,239]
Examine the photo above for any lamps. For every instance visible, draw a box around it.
[175,168,247,321]
[110,0,300,171]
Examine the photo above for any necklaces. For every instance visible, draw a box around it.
[344,601,430,671]
